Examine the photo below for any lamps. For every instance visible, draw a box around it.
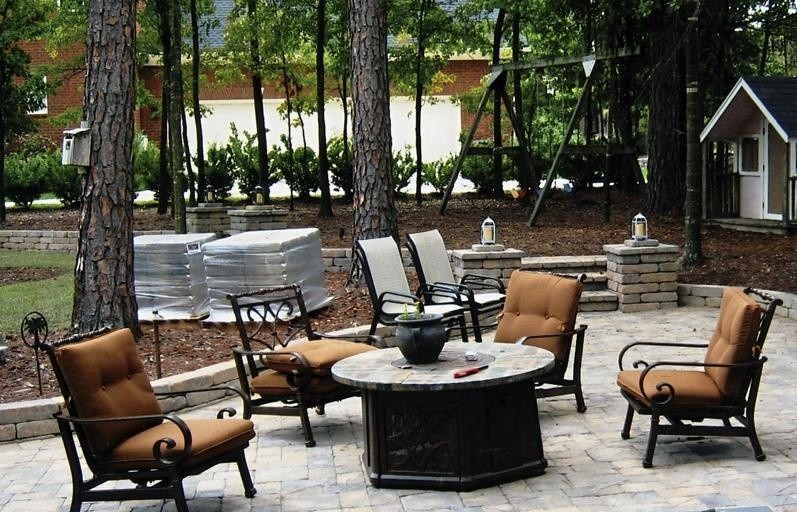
[631,211,647,240]
[480,217,496,245]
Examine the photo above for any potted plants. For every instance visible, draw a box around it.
[394,299,447,364]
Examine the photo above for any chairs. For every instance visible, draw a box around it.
[38,325,258,512]
[613,285,785,471]
[484,265,592,413]
[351,225,506,343]
[225,280,387,452]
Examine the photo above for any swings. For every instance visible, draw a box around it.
[509,70,557,203]
